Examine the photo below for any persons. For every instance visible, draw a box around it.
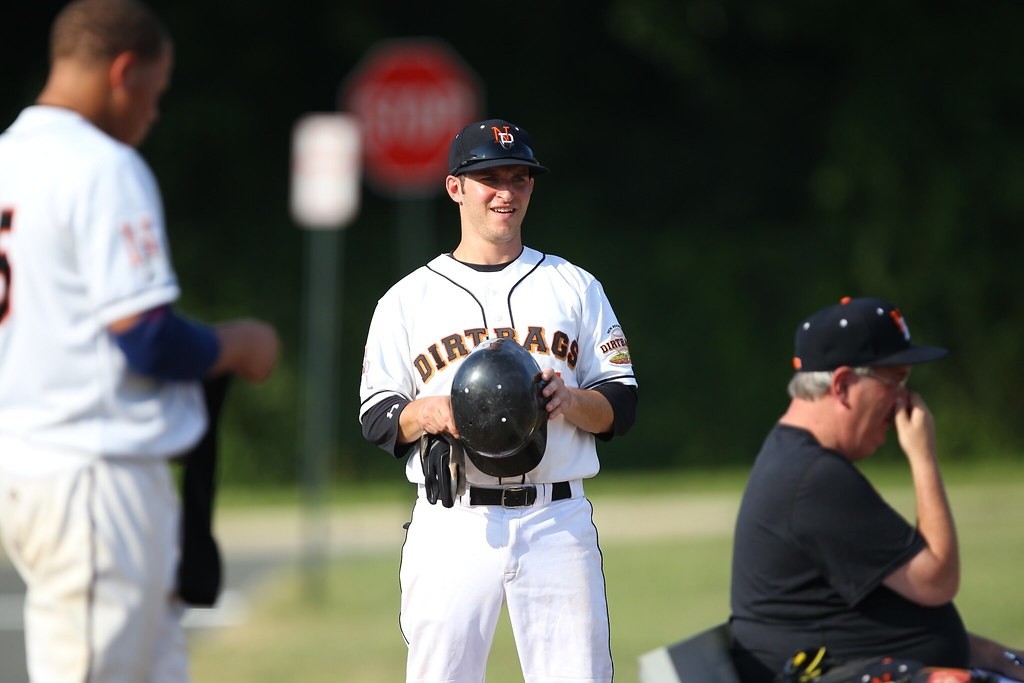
[727,297,1024,683]
[359,117,640,683]
[1,1,279,683]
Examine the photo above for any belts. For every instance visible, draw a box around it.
[460,479,571,508]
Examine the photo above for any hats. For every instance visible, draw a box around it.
[448,119,550,177]
[794,296,954,374]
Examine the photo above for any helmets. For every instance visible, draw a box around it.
[451,338,550,480]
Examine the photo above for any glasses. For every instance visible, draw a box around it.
[860,370,912,390]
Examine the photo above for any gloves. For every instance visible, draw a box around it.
[424,432,466,509]
